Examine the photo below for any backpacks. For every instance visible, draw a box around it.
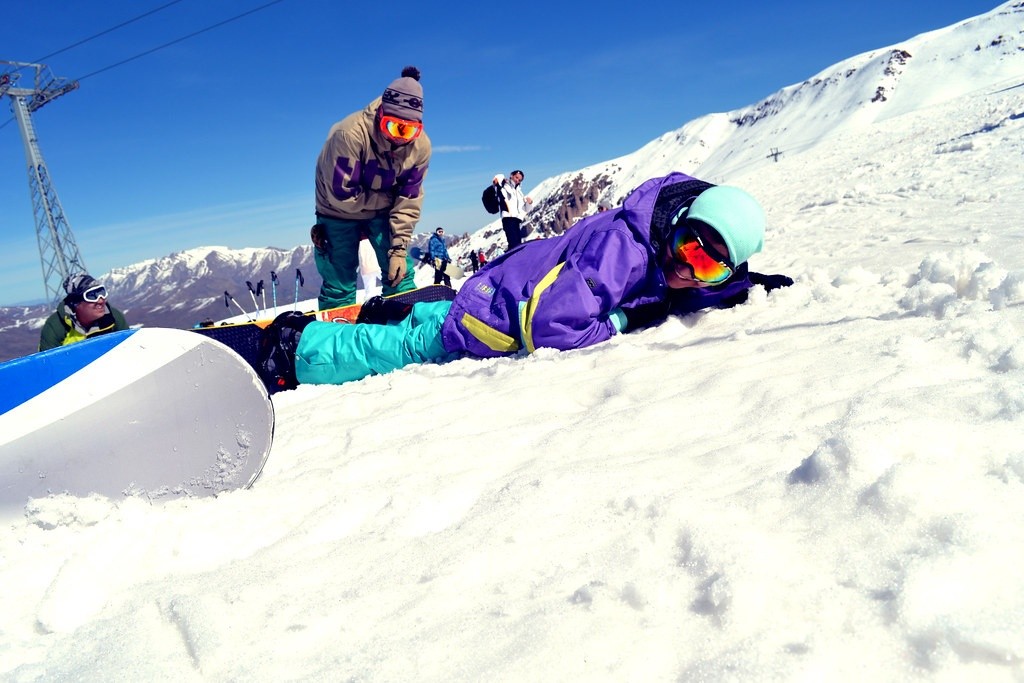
[482,181,499,214]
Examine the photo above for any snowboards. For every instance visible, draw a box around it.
[1,322,279,522]
[410,246,465,281]
[186,284,465,399]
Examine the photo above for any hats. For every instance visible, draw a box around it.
[63,272,96,305]
[382,66,424,121]
[687,185,763,269]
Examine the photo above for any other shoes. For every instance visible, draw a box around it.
[261,311,310,393]
[357,294,386,328]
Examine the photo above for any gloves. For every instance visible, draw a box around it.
[388,248,407,287]
[432,260,436,267]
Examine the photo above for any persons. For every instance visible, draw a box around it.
[492,170,533,251]
[310,76,432,312]
[456,247,488,278]
[254,184,794,396]
[428,227,453,286]
[37,272,130,352]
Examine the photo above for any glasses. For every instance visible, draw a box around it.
[437,230,444,235]
[671,216,734,287]
[83,283,108,304]
[380,114,423,143]
